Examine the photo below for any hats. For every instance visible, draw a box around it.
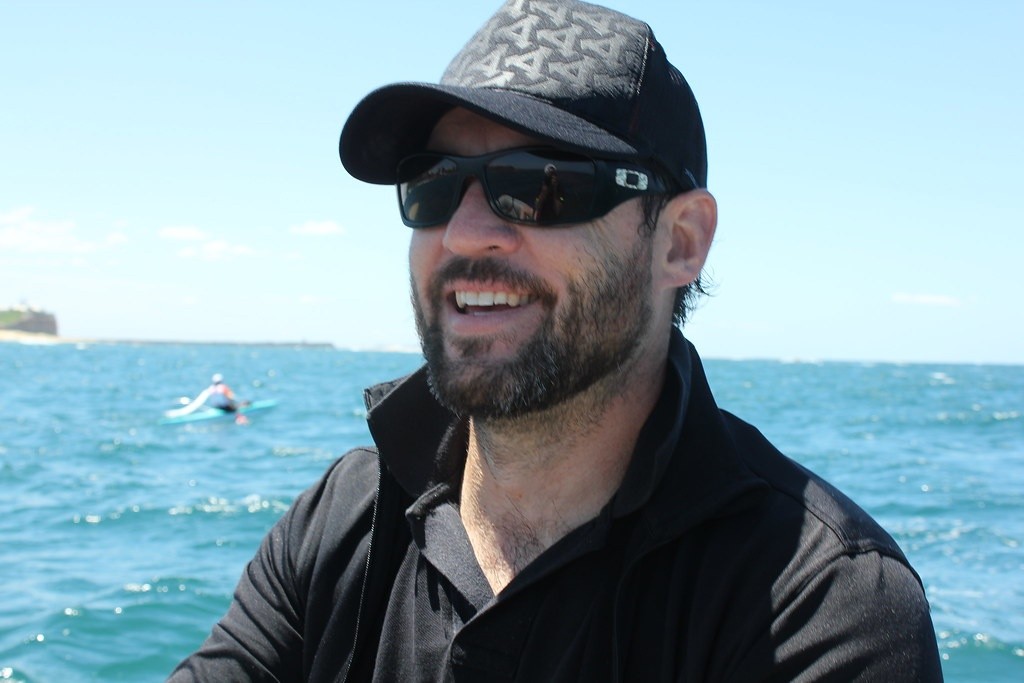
[336,0,710,193]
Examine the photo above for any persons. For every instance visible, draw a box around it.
[163,1,952,683]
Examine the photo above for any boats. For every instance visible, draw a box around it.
[154,394,276,427]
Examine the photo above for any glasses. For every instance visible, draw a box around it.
[393,151,670,230]
